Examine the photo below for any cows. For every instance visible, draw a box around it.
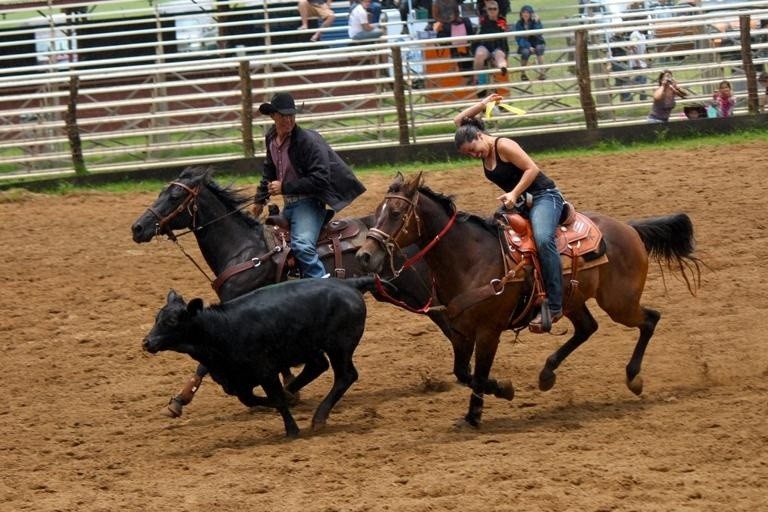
[142,278,367,439]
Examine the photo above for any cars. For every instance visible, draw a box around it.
[578,2,768,64]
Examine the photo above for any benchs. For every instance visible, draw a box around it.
[2,3,513,173]
[560,2,766,103]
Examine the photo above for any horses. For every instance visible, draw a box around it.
[355,171,717,435]
[131,167,452,418]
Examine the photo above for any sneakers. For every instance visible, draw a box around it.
[532,306,564,323]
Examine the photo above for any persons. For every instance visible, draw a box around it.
[251,91,366,279]
[758,71,767,107]
[609,17,633,101]
[578,0,703,24]
[296,0,546,98]
[452,93,563,334]
[710,80,737,118]
[628,30,646,99]
[683,101,709,119]
[645,68,687,123]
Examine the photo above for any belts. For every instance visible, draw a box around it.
[514,192,533,210]
[285,195,309,203]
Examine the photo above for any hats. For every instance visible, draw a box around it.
[258,92,305,115]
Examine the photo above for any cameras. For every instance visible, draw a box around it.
[665,77,672,84]
